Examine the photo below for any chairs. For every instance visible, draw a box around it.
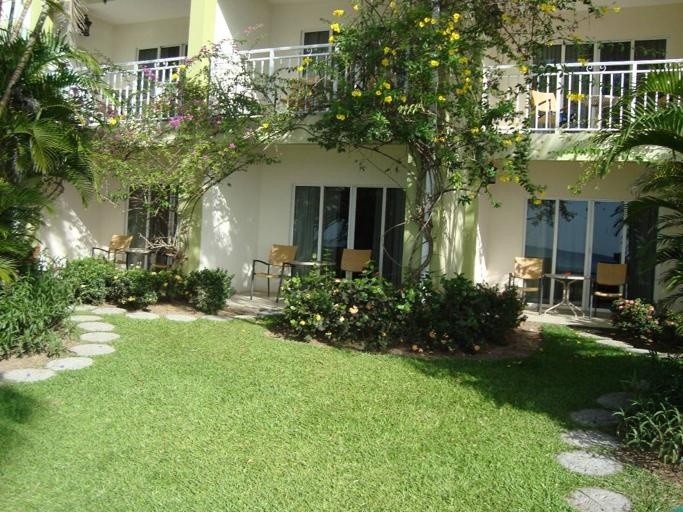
[589,262,627,319]
[509,257,544,315]
[250,244,298,300]
[92,233,134,270]
[334,249,372,284]
[529,90,577,134]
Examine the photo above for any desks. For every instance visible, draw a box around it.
[125,248,152,270]
[542,274,590,320]
[276,261,336,303]
[580,94,620,129]
